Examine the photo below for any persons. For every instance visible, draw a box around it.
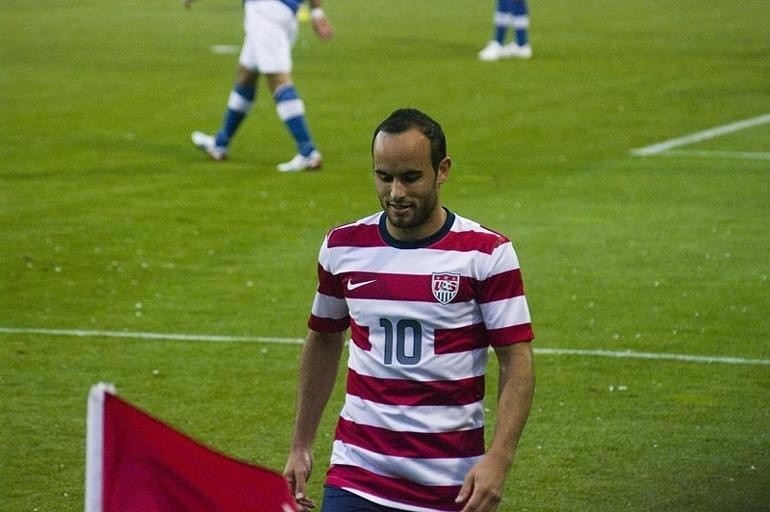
[191,0,333,173]
[284,109,536,512]
[476,0,533,61]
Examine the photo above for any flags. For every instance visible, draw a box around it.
[85,383,304,512]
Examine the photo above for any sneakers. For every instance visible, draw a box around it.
[476,38,534,60]
[189,130,224,161]
[276,150,322,172]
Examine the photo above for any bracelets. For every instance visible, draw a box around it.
[310,6,326,18]
